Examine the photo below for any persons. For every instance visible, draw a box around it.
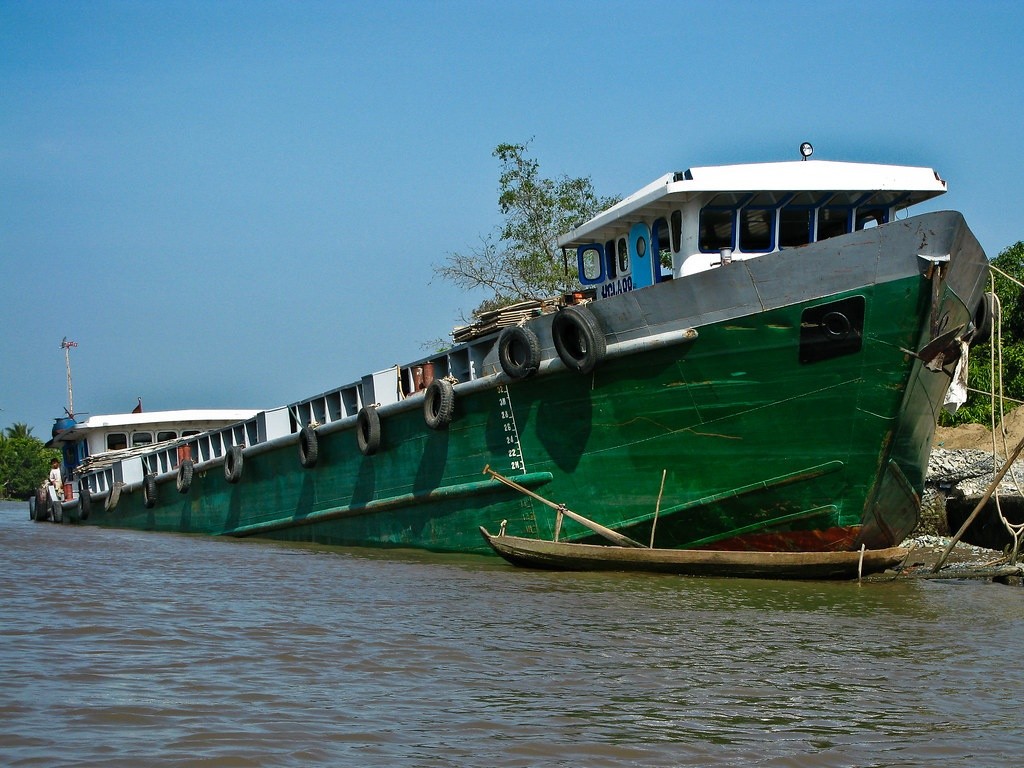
[50,458,64,498]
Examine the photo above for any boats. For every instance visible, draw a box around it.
[27,143,1001,582]
